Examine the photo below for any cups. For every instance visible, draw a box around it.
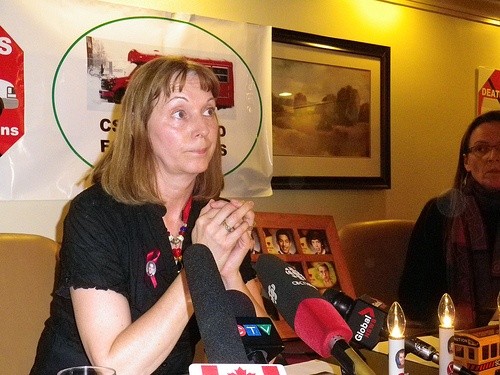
[57,366,116,375]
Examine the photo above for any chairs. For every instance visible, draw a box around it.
[0,233,62,375]
[338,218,416,309]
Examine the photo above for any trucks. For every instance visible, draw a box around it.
[100,49,235,110]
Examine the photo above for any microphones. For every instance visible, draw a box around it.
[181,243,285,375]
[255,253,379,375]
[321,286,435,360]
[224,289,283,375]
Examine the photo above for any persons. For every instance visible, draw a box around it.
[317,263,334,286]
[276,230,293,254]
[251,234,260,254]
[398,111,500,329]
[306,230,328,254]
[28,57,282,375]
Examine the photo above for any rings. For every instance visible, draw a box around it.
[223,221,234,232]
[248,226,253,230]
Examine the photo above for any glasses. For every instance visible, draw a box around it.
[465,145,500,159]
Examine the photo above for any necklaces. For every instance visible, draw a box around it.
[168,194,192,273]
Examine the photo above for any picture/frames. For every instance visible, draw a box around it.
[245,212,357,302]
[271,25,391,189]
